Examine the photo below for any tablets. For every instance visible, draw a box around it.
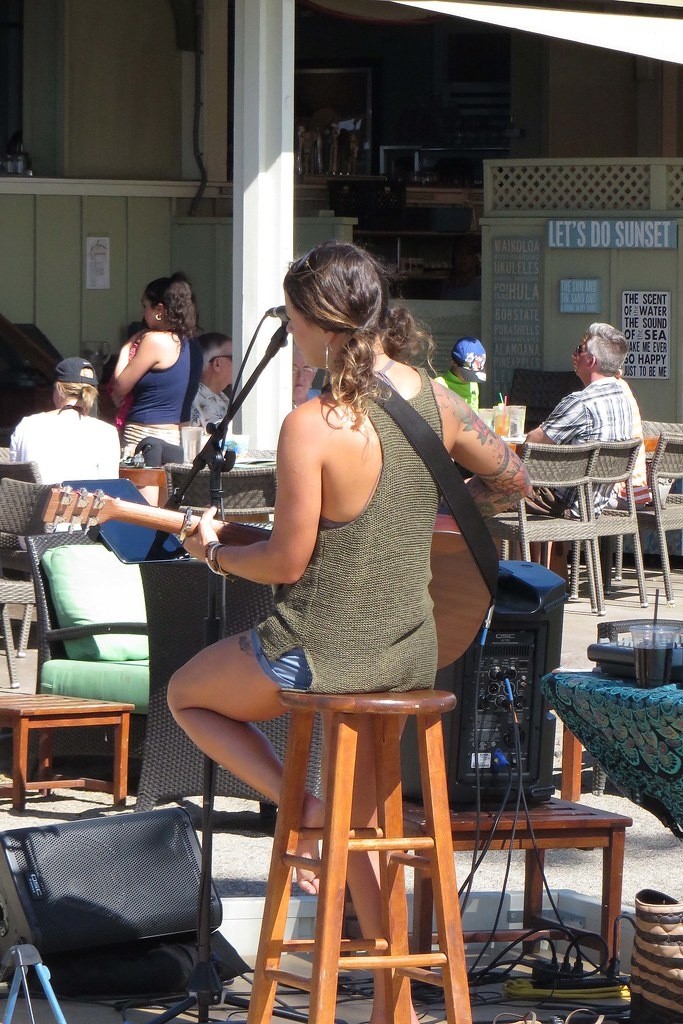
[62,478,192,564]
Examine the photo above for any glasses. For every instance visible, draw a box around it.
[298,248,331,301]
[575,345,593,357]
[292,365,318,374]
[209,354,232,365]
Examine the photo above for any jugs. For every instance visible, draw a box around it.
[80,341,111,381]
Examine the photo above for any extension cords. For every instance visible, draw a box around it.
[530,960,634,991]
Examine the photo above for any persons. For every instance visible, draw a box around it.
[109,272,197,510]
[165,236,533,897]
[292,342,321,411]
[608,366,653,511]
[430,335,489,478]
[8,356,120,484]
[526,321,642,555]
[188,331,234,436]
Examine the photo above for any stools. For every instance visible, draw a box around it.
[243,689,472,1024]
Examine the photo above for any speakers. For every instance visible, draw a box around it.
[399,558,568,810]
[1,805,224,984]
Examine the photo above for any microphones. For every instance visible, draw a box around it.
[266,306,290,319]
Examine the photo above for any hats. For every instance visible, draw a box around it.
[55,357,99,387]
[451,336,488,383]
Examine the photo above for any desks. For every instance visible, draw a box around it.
[402,795,634,978]
[0,693,136,813]
[539,667,683,841]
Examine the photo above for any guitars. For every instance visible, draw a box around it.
[40,482,492,670]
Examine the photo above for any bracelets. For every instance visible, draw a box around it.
[203,540,240,583]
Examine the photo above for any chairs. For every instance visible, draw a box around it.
[0,365,683,813]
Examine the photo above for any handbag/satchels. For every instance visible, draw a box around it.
[630,889,683,1024]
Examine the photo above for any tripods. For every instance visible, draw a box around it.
[115,319,350,1024]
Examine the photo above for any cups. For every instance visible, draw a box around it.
[478,409,493,430]
[493,406,510,437]
[630,625,680,689]
[182,427,202,466]
[509,405,526,438]
